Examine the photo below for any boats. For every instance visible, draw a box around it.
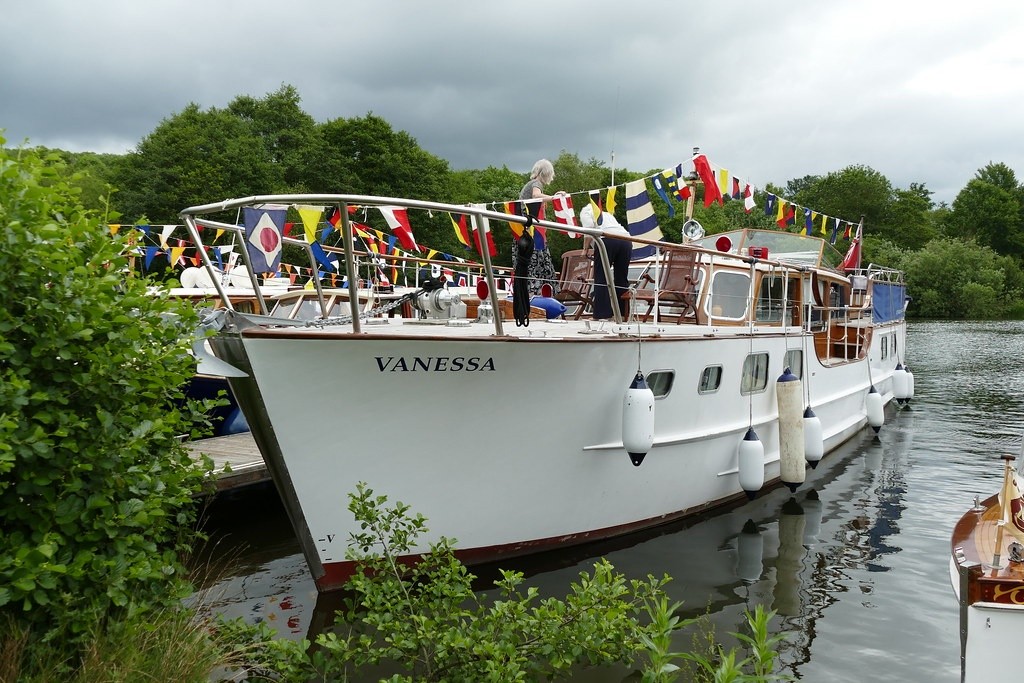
[179,147,913,593]
[103,287,241,442]
[949,446,1024,683]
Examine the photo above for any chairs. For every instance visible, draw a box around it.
[552,249,597,322]
[619,246,705,324]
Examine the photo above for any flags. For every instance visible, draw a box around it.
[837,220,861,274]
[676,164,691,199]
[692,154,723,208]
[448,202,472,250]
[292,203,336,272]
[468,203,497,257]
[552,194,583,238]
[662,170,682,202]
[720,168,853,241]
[998,467,1024,546]
[626,180,665,261]
[353,221,514,291]
[606,187,617,214]
[652,175,674,218]
[378,206,422,254]
[589,191,603,226]
[243,205,289,272]
[504,200,547,250]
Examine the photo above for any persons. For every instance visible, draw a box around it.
[512,159,562,298]
[580,202,633,321]
